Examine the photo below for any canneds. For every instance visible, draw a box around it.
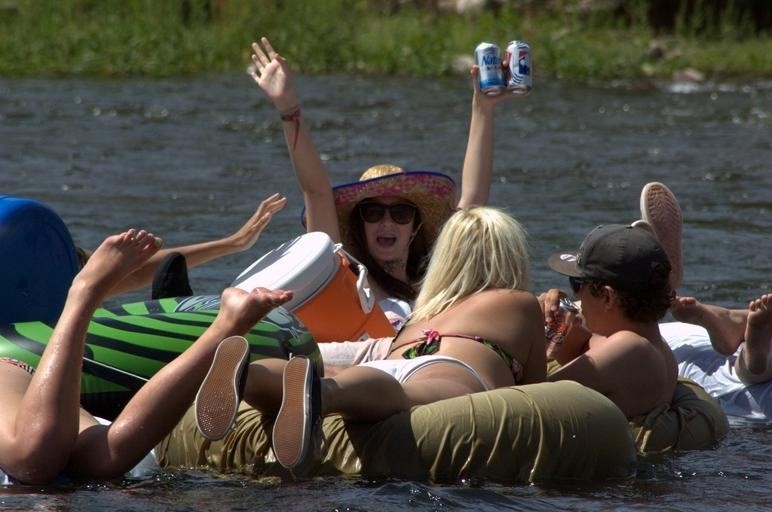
[506,41,533,94]
[474,42,506,97]
[544,297,579,363]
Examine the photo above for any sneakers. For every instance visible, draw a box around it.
[152,252,193,299]
[272,355,321,469]
[632,220,656,237]
[640,182,683,287]
[195,336,250,441]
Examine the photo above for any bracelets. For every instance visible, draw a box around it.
[280,107,302,154]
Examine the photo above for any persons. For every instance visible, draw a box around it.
[192,206,547,469]
[658,291,771,425]
[151,223,678,420]
[77,191,287,305]
[246,37,683,317]
[0,228,293,487]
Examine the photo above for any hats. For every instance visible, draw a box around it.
[302,164,457,252]
[548,225,672,280]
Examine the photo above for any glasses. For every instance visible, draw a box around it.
[569,276,593,294]
[359,200,416,225]
